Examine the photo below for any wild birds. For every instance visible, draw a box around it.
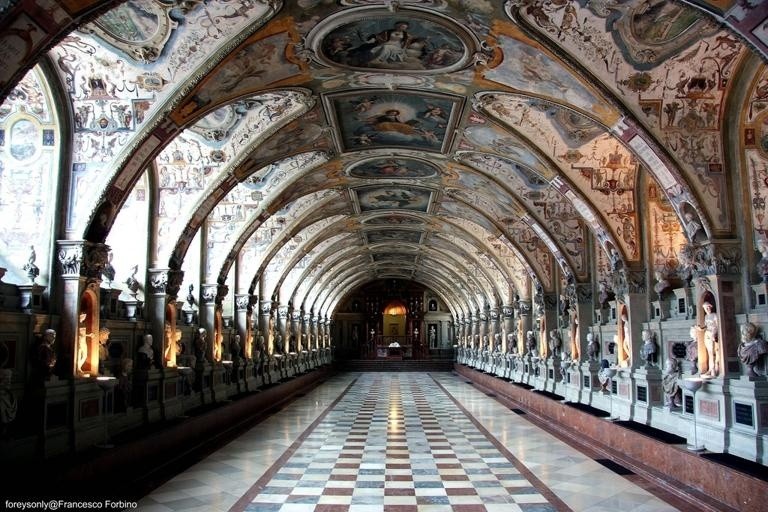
[103,252,116,283]
[125,264,140,294]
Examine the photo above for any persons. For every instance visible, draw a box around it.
[0,327,332,423]
[330,21,455,67]
[455,213,768,406]
[429,326,436,349]
[348,94,448,146]
